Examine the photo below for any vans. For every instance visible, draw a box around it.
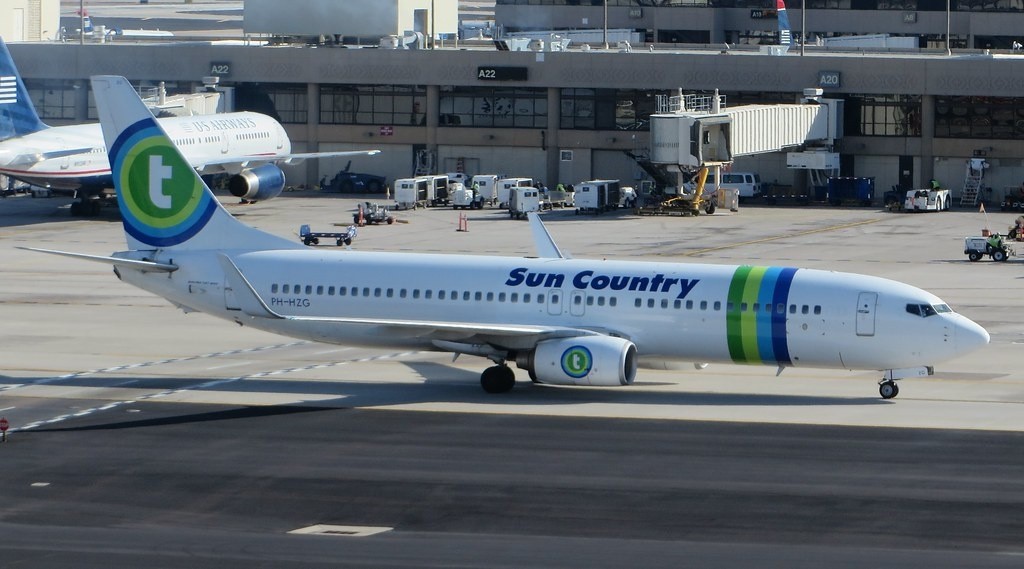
[685,171,762,203]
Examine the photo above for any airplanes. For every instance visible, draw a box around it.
[13,74,991,400]
[0,36,381,217]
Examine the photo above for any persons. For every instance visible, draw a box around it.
[988,233,1001,259]
[471,182,479,198]
[928,177,940,192]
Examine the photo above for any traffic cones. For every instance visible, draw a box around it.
[456,212,469,232]
